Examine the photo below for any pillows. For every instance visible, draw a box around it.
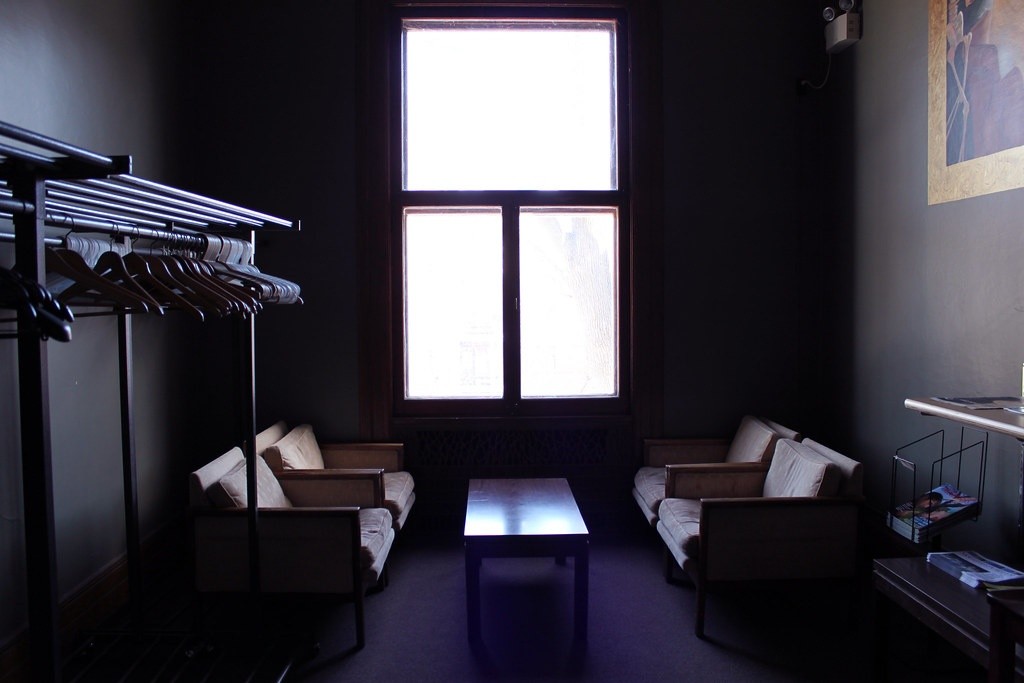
[763,438,841,497]
[265,423,326,470]
[725,411,779,463]
[206,454,293,509]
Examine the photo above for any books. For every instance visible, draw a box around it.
[886,482,982,543]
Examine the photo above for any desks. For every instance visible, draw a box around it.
[905,396,1024,571]
[463,478,592,646]
[870,551,1024,683]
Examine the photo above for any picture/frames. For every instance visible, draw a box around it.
[927,0,1024,206]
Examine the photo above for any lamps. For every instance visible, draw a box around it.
[822,0,860,54]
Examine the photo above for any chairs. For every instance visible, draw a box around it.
[189,447,395,649]
[242,420,416,586]
[662,437,865,639]
[632,417,803,576]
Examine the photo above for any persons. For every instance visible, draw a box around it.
[925,493,978,522]
[888,492,944,518]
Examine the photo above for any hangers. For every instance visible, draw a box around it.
[0,196,305,344]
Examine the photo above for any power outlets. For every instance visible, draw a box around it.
[796,77,820,97]
